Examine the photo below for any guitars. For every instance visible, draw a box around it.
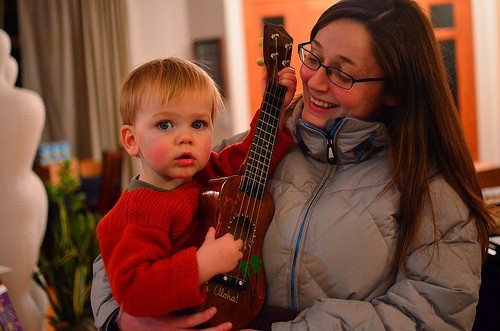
[189,23,295,331]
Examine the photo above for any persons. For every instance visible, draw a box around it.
[89,0,499,331]
[96,55,297,331]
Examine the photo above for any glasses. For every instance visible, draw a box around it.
[298,42,387,90]
